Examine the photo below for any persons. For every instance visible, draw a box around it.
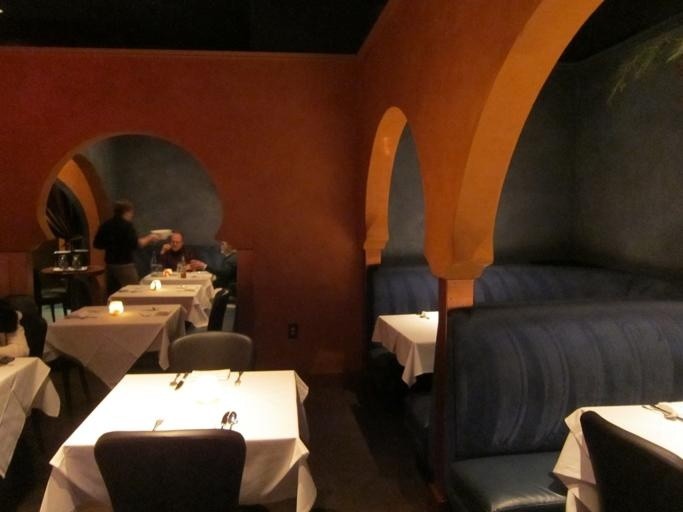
[190,240,238,331]
[0,298,29,358]
[92,198,161,286]
[156,230,197,271]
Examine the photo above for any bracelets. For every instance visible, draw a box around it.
[159,250,164,255]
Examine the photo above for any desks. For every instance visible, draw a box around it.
[0,357,61,480]
[551,400,683,512]
[45,303,188,388]
[107,283,212,327]
[39,369,316,512]
[370,310,439,389]
[140,271,217,297]
[40,265,105,309]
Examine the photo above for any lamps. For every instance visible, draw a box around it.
[109,301,124,313]
[150,280,161,291]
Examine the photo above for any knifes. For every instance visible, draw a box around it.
[175,372,189,391]
[221,413,229,429]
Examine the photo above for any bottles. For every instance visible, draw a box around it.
[180,254,187,278]
[150,250,158,277]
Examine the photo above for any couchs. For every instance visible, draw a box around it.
[370,263,683,382]
[446,300,683,512]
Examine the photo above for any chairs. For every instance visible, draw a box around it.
[579,403,683,511]
[167,332,255,372]
[2,295,93,403]
[206,288,230,330]
[34,269,70,322]
[94,431,247,512]
[20,315,48,455]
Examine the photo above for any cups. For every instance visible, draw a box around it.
[158,264,163,277]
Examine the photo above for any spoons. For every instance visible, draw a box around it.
[228,411,237,431]
